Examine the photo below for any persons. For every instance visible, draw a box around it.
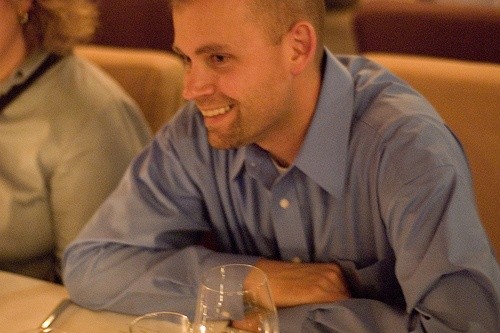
[0,0,155,283]
[62,0,500,333]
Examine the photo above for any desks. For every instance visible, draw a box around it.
[0,271,193,333]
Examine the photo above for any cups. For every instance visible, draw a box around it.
[128,311,191,333]
[191,263,280,333]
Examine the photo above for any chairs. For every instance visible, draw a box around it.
[71,0,500,264]
